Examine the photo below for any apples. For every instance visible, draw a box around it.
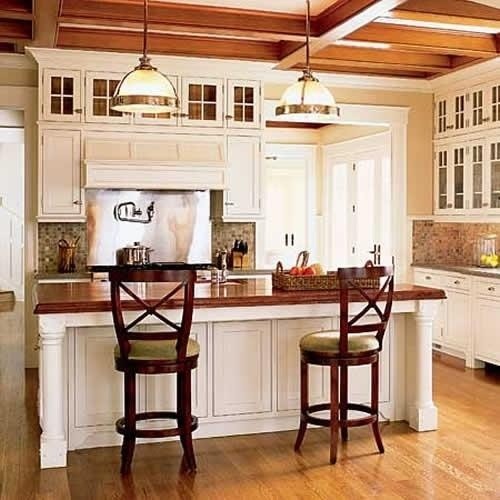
[290,264,324,276]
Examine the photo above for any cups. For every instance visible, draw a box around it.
[59,248,77,272]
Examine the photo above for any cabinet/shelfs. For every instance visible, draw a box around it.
[413,269,468,366]
[432,71,500,223]
[469,275,500,369]
[37,59,261,222]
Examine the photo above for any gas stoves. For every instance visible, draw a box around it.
[111,261,191,271]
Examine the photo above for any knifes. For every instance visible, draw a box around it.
[234,239,248,254]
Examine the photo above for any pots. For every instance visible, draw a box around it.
[122,243,154,267]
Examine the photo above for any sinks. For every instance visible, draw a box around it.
[211,278,246,287]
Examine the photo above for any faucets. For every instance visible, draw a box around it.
[214,248,228,282]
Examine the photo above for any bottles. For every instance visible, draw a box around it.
[220,252,228,270]
[211,267,219,284]
[479,233,496,268]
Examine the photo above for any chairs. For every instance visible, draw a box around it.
[294,266,394,464]
[107,268,200,474]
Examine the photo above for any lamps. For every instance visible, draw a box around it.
[110,0,179,114]
[276,0,340,121]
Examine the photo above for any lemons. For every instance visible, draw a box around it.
[480,254,500,266]
